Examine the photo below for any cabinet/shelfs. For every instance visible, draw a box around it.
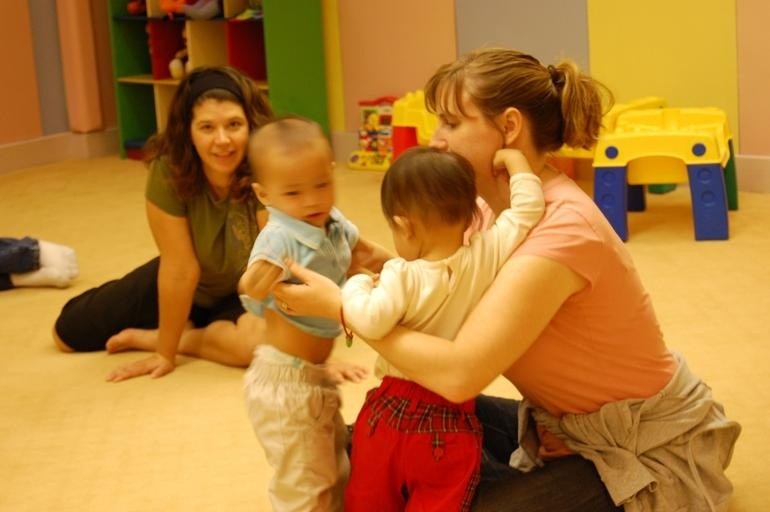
[106,0,331,163]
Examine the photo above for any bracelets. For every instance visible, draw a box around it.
[338,298,353,348]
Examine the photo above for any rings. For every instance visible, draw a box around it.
[282,300,289,311]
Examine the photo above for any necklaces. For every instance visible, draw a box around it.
[209,174,233,192]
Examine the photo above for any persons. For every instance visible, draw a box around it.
[1,231,84,289]
[237,114,391,512]
[52,64,367,384]
[272,44,743,512]
[341,144,545,512]
